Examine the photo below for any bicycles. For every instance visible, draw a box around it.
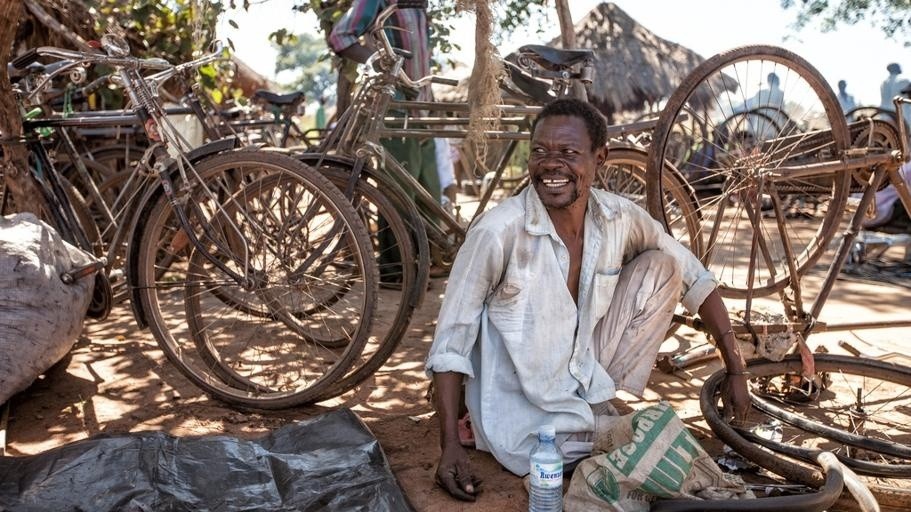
[642,43,910,366]
[0,0,673,349]
[183,0,706,409]
[0,33,379,409]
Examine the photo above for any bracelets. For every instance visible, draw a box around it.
[720,370,754,377]
[714,327,738,350]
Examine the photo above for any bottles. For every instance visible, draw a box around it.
[526,425,564,512]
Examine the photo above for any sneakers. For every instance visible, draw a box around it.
[378,259,434,294]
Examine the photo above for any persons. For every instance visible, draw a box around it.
[419,97,754,503]
[430,109,458,203]
[734,73,785,137]
[879,63,910,129]
[860,161,910,229]
[834,80,854,124]
[336,58,372,259]
[329,1,441,290]
